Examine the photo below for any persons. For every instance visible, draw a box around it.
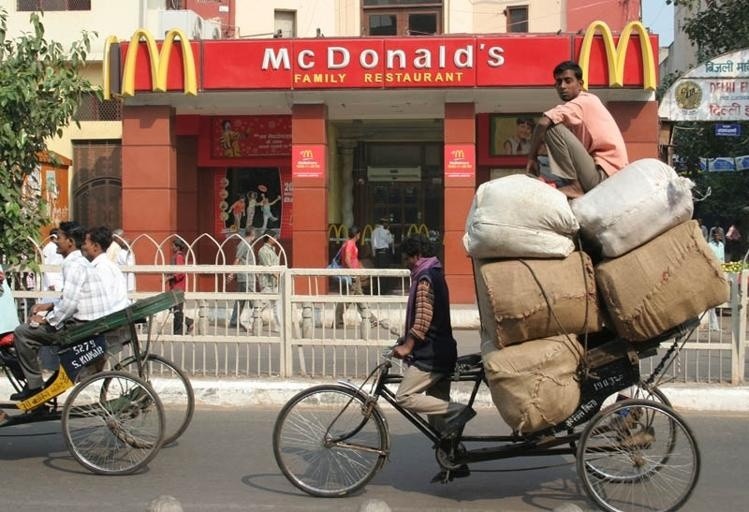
[525,61,630,200]
[159,239,195,334]
[260,193,281,228]
[503,117,537,154]
[332,224,386,329]
[219,120,241,157]
[226,225,257,327]
[698,217,743,266]
[391,233,478,484]
[0,219,139,427]
[228,194,246,228]
[256,230,286,333]
[245,191,264,228]
[371,217,395,293]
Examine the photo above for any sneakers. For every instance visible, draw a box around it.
[229,322,246,331]
[431,465,469,484]
[437,404,477,442]
[187,319,194,332]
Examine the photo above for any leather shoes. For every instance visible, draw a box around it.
[9,383,42,401]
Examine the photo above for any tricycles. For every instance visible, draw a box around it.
[1,330,195,477]
[273,338,701,512]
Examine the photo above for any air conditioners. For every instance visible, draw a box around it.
[205,19,221,40]
[163,9,205,40]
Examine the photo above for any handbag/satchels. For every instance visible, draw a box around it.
[325,262,353,287]
